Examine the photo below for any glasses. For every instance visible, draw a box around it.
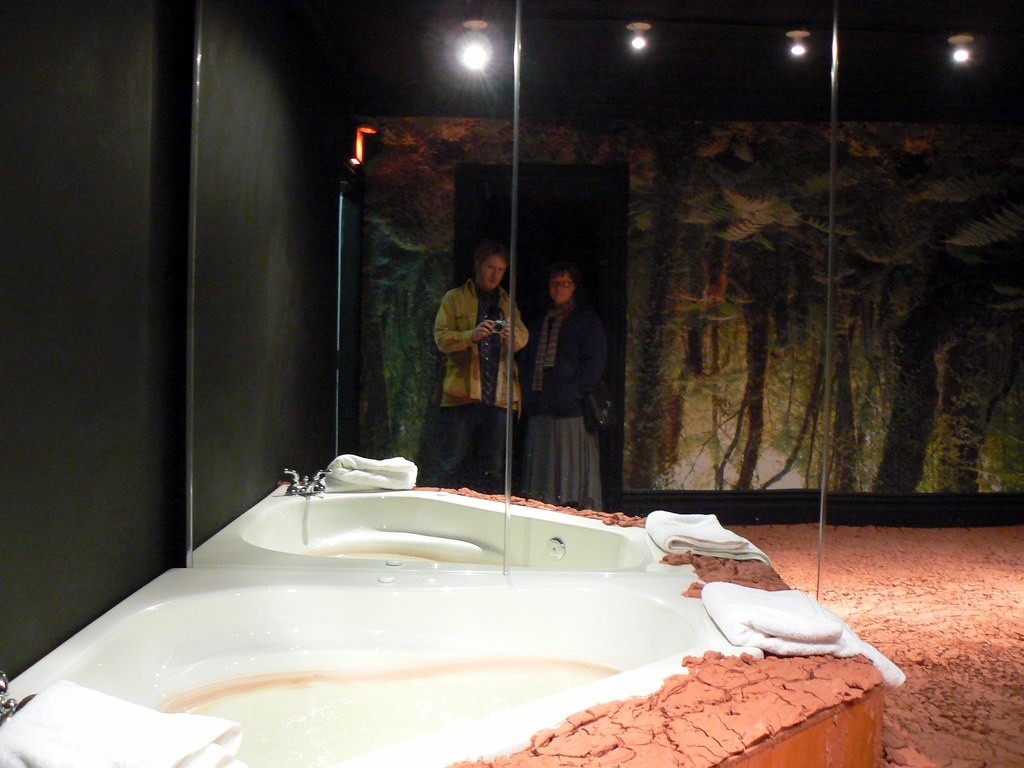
[549,281,573,287]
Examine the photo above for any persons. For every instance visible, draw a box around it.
[421,240,529,495]
[515,263,606,512]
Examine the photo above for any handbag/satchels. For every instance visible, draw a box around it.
[583,368,621,431]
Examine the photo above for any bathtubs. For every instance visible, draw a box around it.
[0,568,884,768]
[187,481,790,578]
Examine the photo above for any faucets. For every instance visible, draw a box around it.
[305,480,325,494]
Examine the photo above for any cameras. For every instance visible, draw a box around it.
[490,321,504,334]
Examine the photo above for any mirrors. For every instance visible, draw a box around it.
[186,0,518,575]
[815,0,1024,768]
[508,0,837,601]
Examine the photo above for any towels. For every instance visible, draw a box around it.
[322,454,418,493]
[701,581,863,656]
[0,681,247,767]
[645,510,772,566]
